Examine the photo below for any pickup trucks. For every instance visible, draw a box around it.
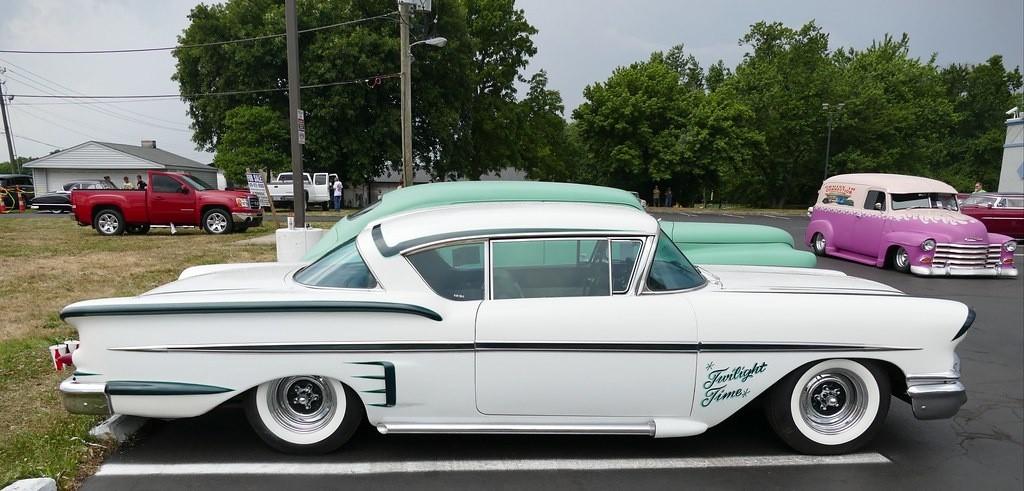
[266,172,338,211]
[70,170,264,235]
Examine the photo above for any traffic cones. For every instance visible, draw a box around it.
[0,198,6,211]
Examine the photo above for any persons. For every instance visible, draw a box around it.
[104,175,118,189]
[136,175,147,190]
[653,186,661,207]
[328,178,343,212]
[665,187,672,207]
[972,182,987,194]
[123,176,134,190]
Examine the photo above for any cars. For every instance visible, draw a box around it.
[804,174,1019,277]
[49,180,976,454]
[0,174,35,210]
[28,180,123,214]
[945,193,1024,239]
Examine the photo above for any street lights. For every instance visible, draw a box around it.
[822,102,846,180]
[400,37,448,188]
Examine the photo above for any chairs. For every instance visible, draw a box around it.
[407,250,525,300]
